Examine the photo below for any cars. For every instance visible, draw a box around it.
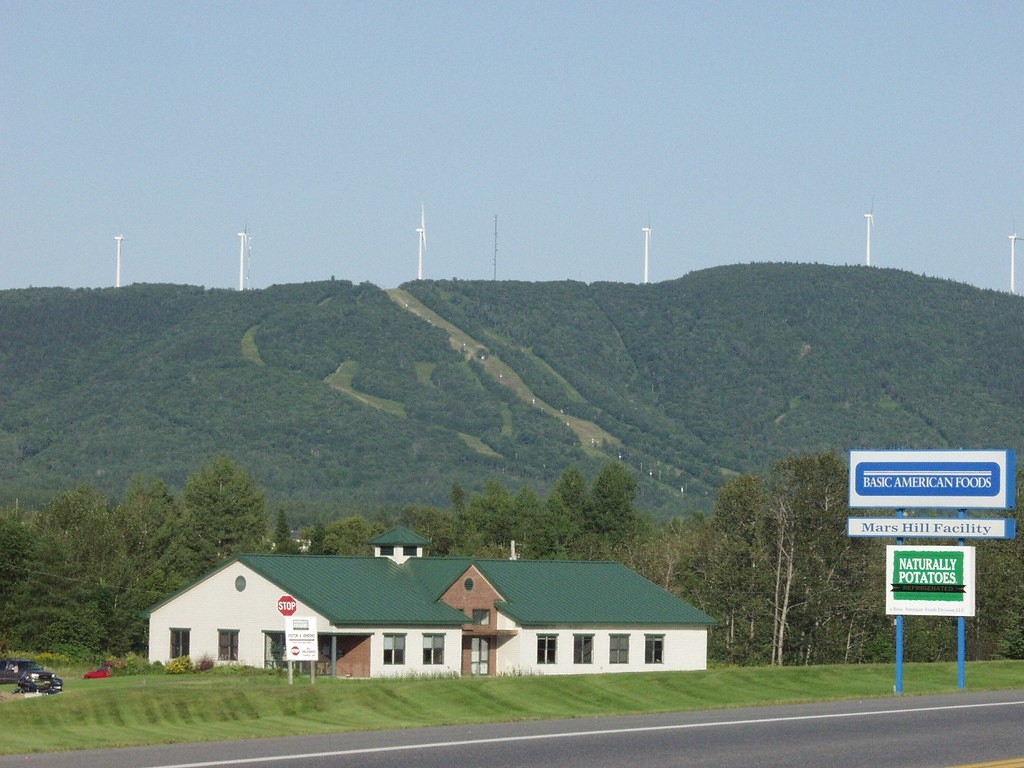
[0,658,44,685]
[82,661,115,680]
[12,670,64,695]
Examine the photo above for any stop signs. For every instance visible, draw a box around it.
[277,595,297,617]
